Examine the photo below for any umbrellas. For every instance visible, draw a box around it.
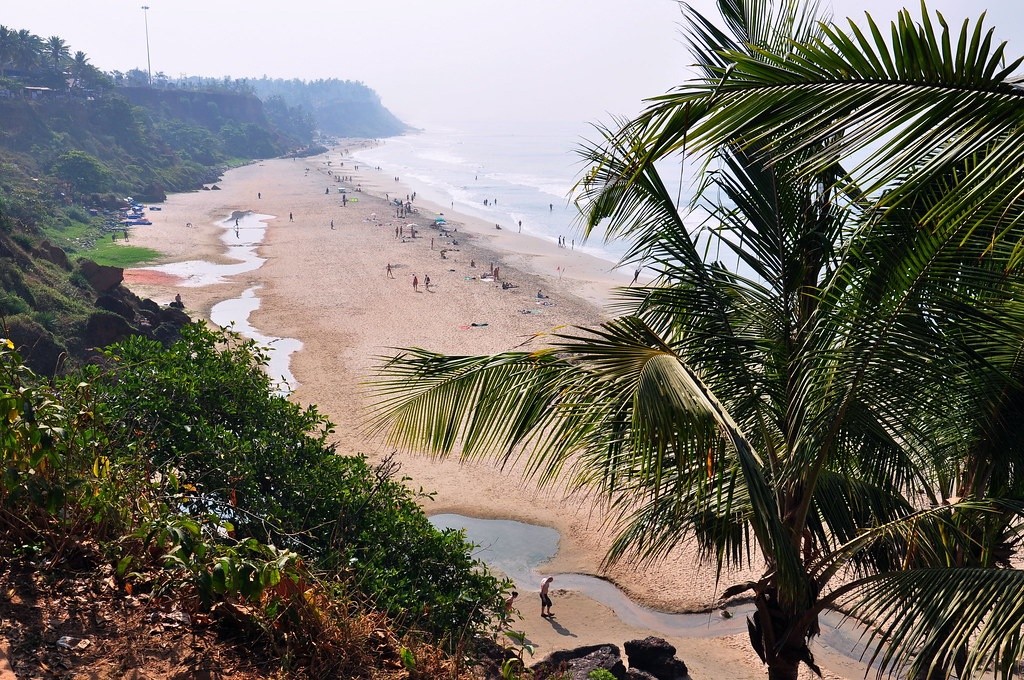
[436,222,445,225]
[434,217,446,222]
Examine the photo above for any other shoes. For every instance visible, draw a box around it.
[548,613,555,617]
[541,614,548,617]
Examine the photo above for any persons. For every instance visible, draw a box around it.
[424,275,430,290]
[233,218,238,228]
[496,224,501,230]
[550,204,553,209]
[558,235,574,249]
[540,576,555,617]
[471,260,518,289]
[431,221,458,259]
[538,290,542,298]
[412,274,418,291]
[175,294,181,307]
[331,220,334,229]
[505,591,518,618]
[396,225,415,238]
[323,160,361,206]
[289,212,293,222]
[111,231,129,241]
[386,264,393,278]
[519,221,522,226]
[386,191,416,218]
[258,193,260,198]
[484,198,497,206]
